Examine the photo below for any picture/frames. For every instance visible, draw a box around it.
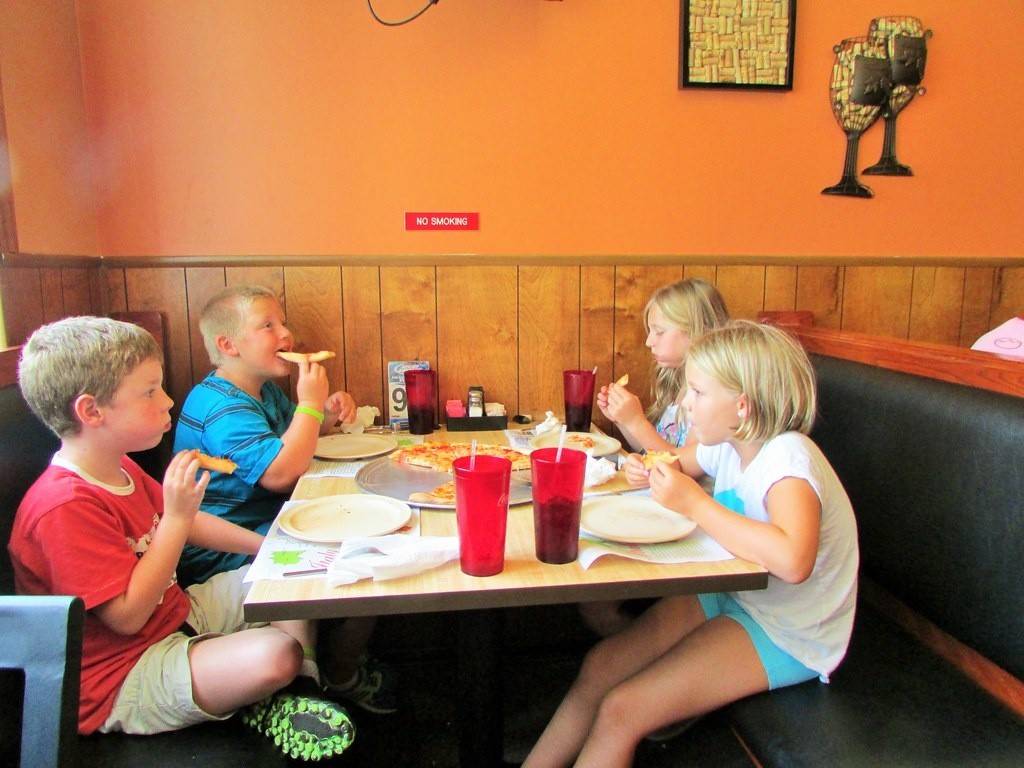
[678,0,797,94]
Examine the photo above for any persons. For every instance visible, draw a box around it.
[177,283,402,714]
[520,322,860,768]
[577,279,730,740]
[7,314,358,761]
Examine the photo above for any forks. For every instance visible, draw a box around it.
[364,421,410,432]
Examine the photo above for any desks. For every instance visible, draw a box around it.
[242,416,768,768]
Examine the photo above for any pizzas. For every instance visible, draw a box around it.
[384,441,535,506]
[276,349,337,363]
[191,453,239,476]
[571,435,594,449]
[641,449,683,470]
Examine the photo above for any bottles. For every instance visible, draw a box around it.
[468,389,483,418]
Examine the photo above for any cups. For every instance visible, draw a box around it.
[452,454,512,577]
[404,369,437,435]
[561,368,596,433]
[529,447,588,564]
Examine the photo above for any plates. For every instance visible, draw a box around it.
[579,494,698,545]
[278,492,412,544]
[313,433,398,462]
[529,431,622,458]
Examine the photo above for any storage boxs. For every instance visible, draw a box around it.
[447,415,508,431]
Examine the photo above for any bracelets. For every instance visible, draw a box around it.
[294,405,325,425]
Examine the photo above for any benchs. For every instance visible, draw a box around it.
[0,313,279,768]
[620,310,1024,768]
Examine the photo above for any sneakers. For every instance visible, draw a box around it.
[241,672,358,763]
[322,650,403,714]
